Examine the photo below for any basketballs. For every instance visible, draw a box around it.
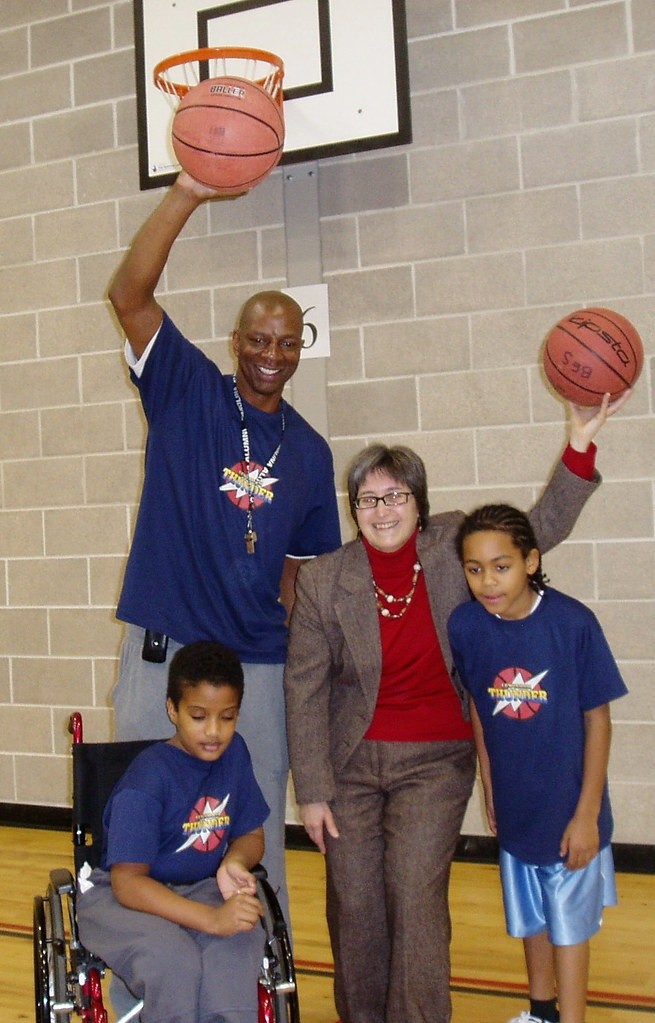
[171,76,285,192]
[542,309,644,406]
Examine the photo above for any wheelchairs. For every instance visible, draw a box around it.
[32,712,301,1023]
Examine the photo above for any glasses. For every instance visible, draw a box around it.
[354,492,414,509]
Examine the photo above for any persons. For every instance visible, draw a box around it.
[445,501,631,1023]
[76,638,272,1023]
[283,383,637,1023]
[106,170,343,1023]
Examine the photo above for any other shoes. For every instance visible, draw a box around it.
[510,1010,551,1023]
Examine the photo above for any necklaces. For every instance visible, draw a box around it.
[372,562,421,618]
